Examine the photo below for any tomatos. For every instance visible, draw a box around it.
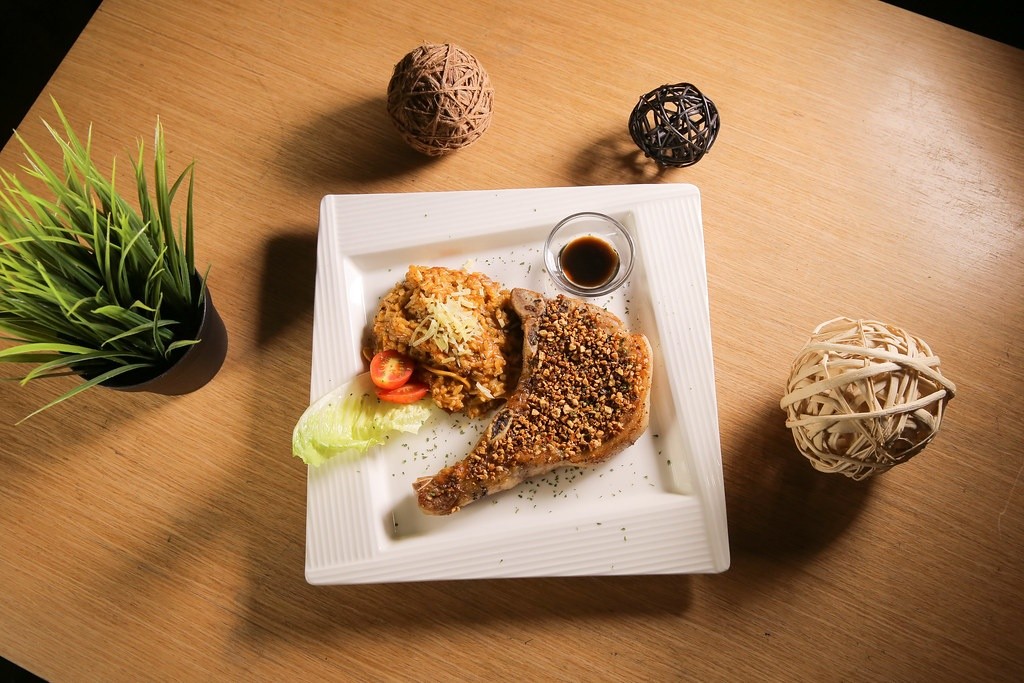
[375,376,429,404]
[370,349,414,389]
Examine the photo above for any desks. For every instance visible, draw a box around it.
[0,0,1024,683]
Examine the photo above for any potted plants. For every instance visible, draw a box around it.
[0,94,229,426]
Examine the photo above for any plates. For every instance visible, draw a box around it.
[304,184,732,586]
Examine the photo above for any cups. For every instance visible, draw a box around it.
[543,211,636,298]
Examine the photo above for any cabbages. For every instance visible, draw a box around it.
[292,371,435,467]
